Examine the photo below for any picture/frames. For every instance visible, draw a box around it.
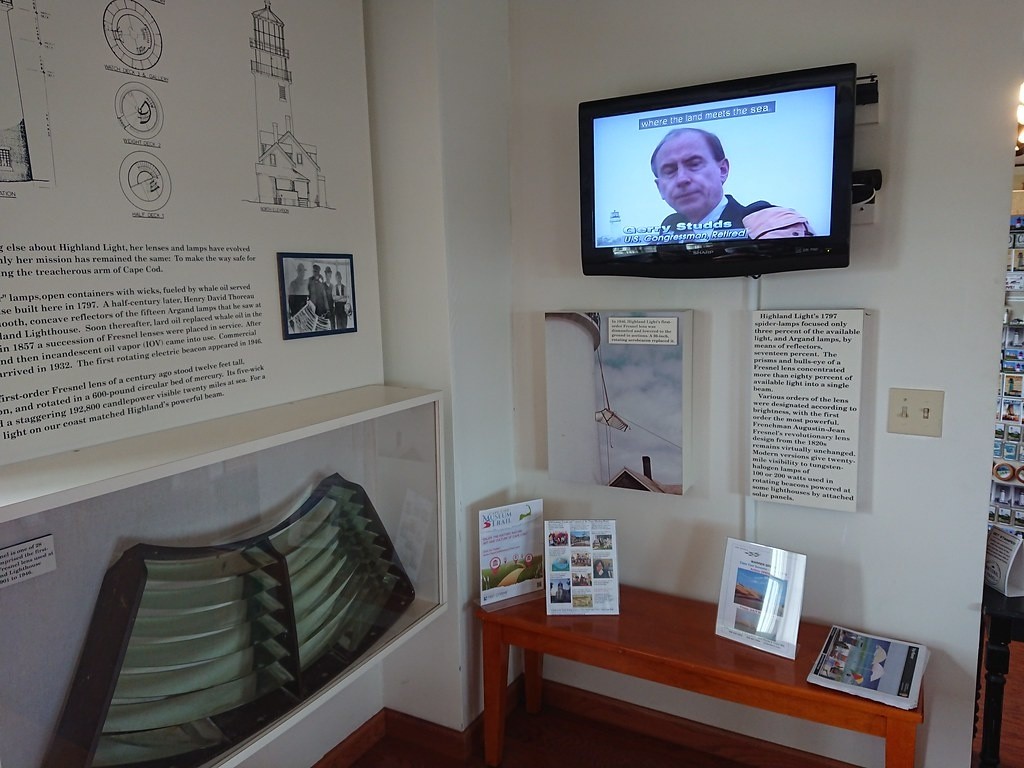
[276,252,358,342]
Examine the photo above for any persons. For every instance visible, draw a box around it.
[650,128,781,245]
[593,559,610,578]
[289,263,350,330]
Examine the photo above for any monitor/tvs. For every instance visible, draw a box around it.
[577,62,858,279]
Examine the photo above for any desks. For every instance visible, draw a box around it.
[971,585,1024,767]
[475,582,923,768]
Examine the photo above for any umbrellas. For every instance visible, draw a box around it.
[843,645,887,681]
